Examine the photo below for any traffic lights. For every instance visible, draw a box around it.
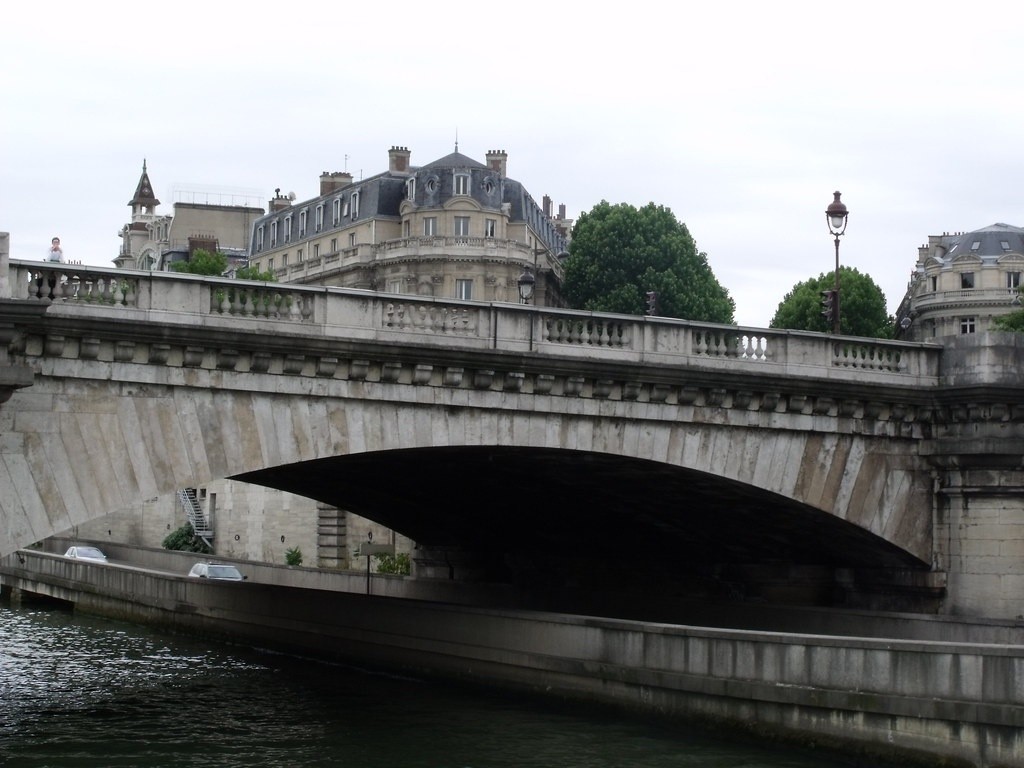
[820,289,838,323]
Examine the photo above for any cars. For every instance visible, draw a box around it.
[190,562,249,584]
[64,546,108,565]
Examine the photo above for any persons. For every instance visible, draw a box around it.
[43,237,64,263]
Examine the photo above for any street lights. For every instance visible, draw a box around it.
[824,191,849,334]
[517,263,537,306]
